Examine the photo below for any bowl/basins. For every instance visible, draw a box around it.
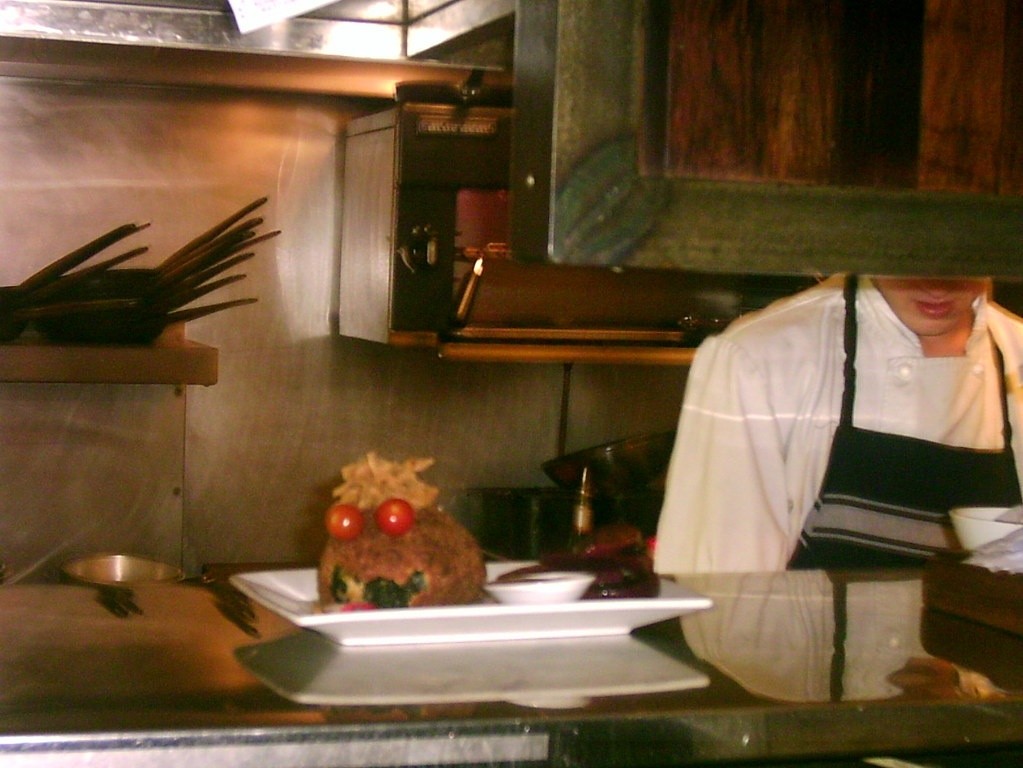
[948,507,1023,573]
[482,572,597,604]
[61,552,184,589]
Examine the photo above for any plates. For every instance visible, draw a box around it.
[230,561,714,644]
[232,632,710,707]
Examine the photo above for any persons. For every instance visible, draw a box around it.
[653,277,1023,574]
[658,577,1003,704]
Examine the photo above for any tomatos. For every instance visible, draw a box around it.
[375,498,414,537]
[324,502,363,541]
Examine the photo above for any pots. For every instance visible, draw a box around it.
[397,63,492,108]
[0,195,282,347]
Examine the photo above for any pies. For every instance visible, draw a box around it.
[312,506,486,609]
